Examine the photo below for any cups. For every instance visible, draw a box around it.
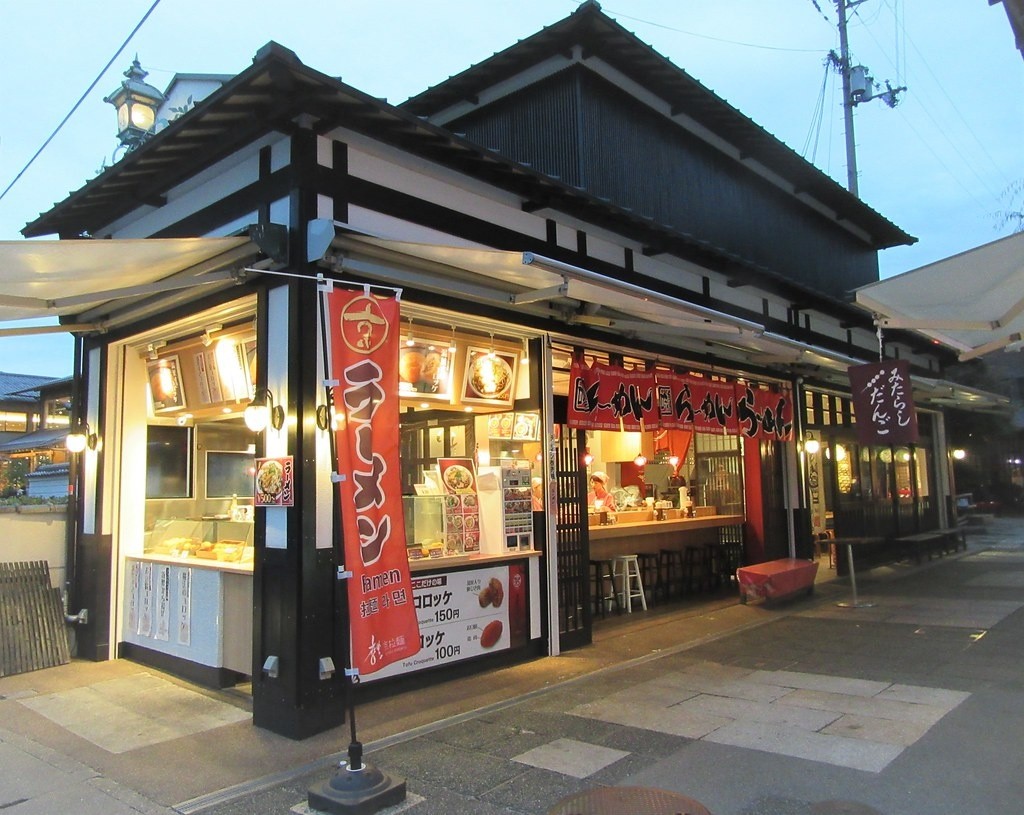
[686,505,692,517]
[600,512,607,525]
[656,509,663,521]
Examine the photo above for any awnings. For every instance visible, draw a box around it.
[0,428,70,452]
[0,235,254,338]
[841,229,1024,362]
[351,227,1011,413]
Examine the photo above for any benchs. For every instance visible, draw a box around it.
[735,557,817,606]
[895,527,971,563]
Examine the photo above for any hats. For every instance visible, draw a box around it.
[593,471,609,484]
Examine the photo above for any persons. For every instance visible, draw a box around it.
[531,477,544,511]
[587,471,617,513]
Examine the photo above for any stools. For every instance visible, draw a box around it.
[589,544,738,619]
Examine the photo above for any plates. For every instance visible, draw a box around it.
[256,461,286,500]
[452,516,463,528]
[446,495,460,509]
[465,516,475,528]
[443,465,474,492]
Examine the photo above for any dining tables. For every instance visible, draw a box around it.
[817,537,880,608]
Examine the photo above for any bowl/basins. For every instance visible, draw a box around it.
[469,355,512,398]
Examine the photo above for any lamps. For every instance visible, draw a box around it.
[448,322,457,353]
[405,314,415,347]
[65,417,103,453]
[520,334,530,363]
[801,430,819,455]
[635,452,647,467]
[947,442,966,461]
[487,328,495,360]
[200,323,223,347]
[103,53,166,155]
[317,386,337,431]
[244,385,285,434]
[824,443,846,462]
[149,340,167,359]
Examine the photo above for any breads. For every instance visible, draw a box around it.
[398,352,441,385]
